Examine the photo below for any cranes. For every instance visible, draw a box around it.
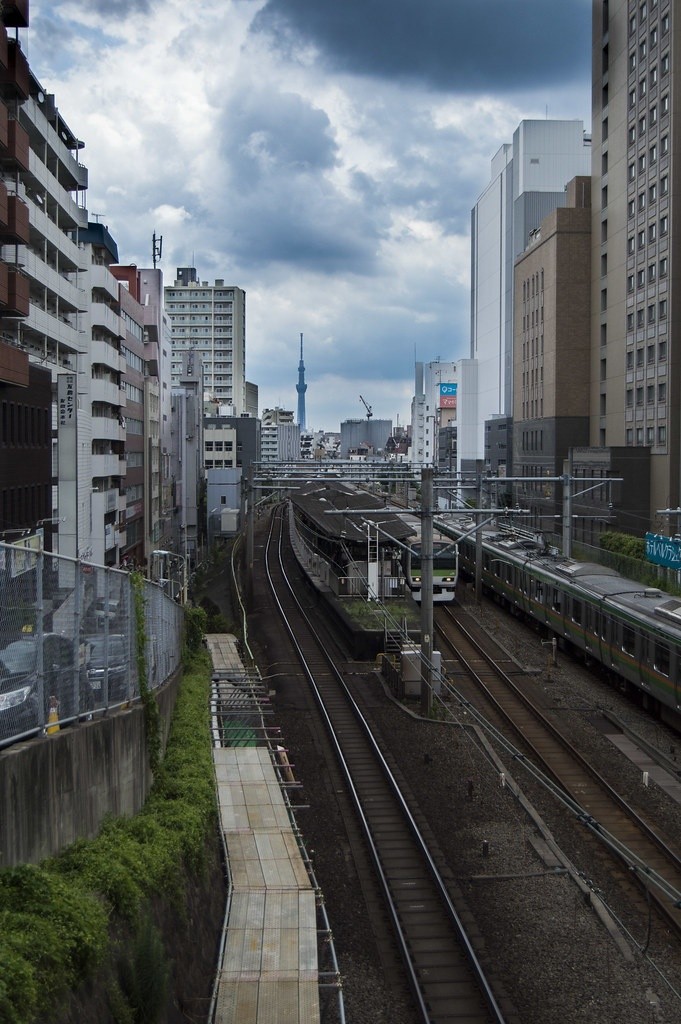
[359,395,372,421]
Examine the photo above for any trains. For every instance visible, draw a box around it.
[341,480,458,601]
[350,480,681,715]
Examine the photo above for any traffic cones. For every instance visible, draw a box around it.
[47,697,60,735]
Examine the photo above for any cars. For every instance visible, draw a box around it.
[78,634,128,701]
[0,631,95,746]
[89,597,120,630]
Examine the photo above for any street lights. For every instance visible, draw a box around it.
[160,578,183,606]
[153,549,187,606]
